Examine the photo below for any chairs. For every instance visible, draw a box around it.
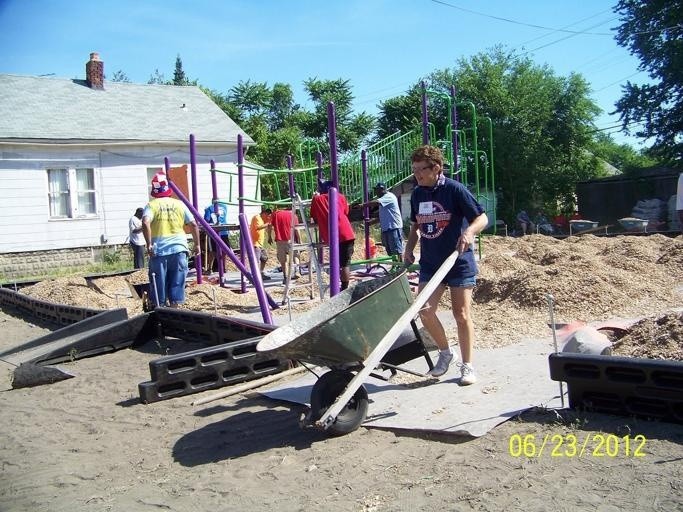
[494,219,508,237]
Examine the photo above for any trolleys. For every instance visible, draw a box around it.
[255,246,460,437]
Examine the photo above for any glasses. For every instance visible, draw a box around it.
[411,165,431,172]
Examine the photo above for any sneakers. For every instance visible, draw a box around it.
[202,270,212,275]
[430,348,453,377]
[261,272,271,280]
[456,362,474,385]
[294,271,300,278]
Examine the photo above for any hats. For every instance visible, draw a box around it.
[372,183,386,190]
[150,171,172,197]
[317,178,333,192]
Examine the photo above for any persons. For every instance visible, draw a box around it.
[267,205,302,284]
[309,179,355,292]
[400,145,488,385]
[202,196,232,276]
[352,181,404,274]
[249,208,274,280]
[128,207,146,270]
[139,171,198,310]
[514,208,587,236]
